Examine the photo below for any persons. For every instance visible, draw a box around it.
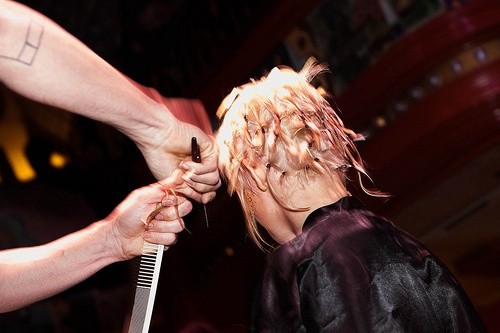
[216,58,482,333]
[1,1,222,313]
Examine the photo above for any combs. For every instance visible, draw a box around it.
[128,241,163,333]
